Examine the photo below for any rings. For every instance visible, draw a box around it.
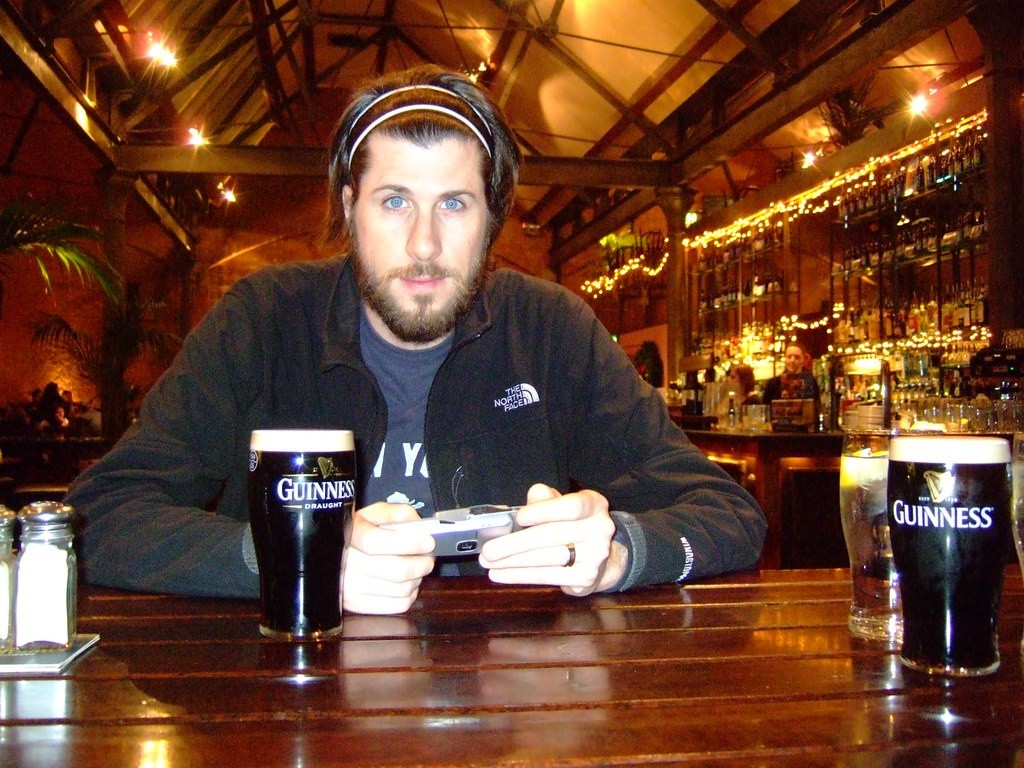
[563,543,575,568]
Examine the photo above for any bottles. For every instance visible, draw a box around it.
[742,404,770,432]
[15,501,78,652]
[727,399,735,427]
[836,125,988,366]
[0,505,17,654]
[698,270,784,311]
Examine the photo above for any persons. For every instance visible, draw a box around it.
[61,63,768,615]
[0,382,80,482]
[728,341,820,434]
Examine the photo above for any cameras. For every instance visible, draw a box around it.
[381,501,536,557]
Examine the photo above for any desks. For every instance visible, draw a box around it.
[0,568,1024,768]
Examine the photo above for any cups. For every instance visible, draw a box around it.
[898,396,1024,433]
[887,435,1013,674]
[1011,430,1024,664]
[839,429,944,643]
[247,427,357,644]
